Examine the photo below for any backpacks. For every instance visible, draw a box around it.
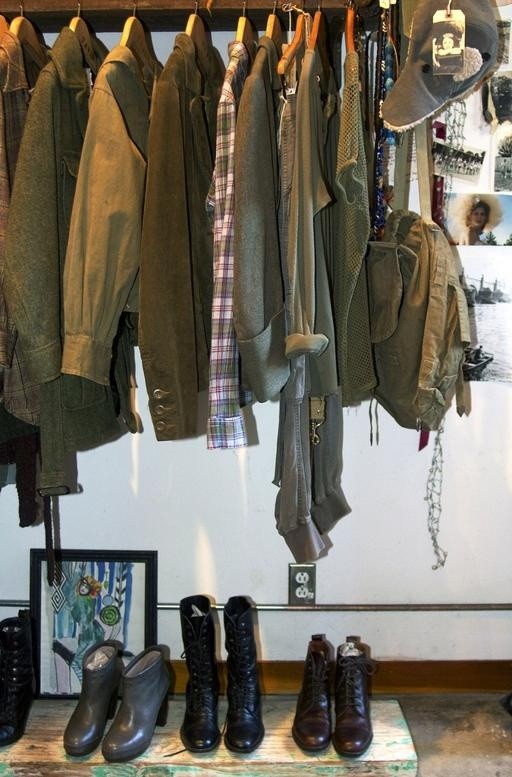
[368,210,477,430]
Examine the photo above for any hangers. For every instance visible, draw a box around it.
[180,2,218,72]
[68,2,98,75]
[11,3,46,64]
[120,2,157,76]
[235,2,258,64]
[266,1,286,61]
[300,1,335,76]
[344,2,360,54]
[278,1,306,76]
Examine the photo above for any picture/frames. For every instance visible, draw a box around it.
[29,548,158,701]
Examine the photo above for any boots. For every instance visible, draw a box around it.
[0,616,34,744]
[64,641,175,762]
[291,634,332,751]
[333,636,373,757]
[224,595,264,752]
[179,595,222,753]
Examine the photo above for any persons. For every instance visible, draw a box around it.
[71,574,104,685]
[433,154,481,175]
[471,346,484,360]
[458,202,490,245]
[499,159,508,178]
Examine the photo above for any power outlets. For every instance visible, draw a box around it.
[288,563,316,605]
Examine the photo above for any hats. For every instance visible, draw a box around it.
[379,0,498,134]
[453,195,502,232]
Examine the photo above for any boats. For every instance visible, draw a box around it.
[462,347,497,377]
